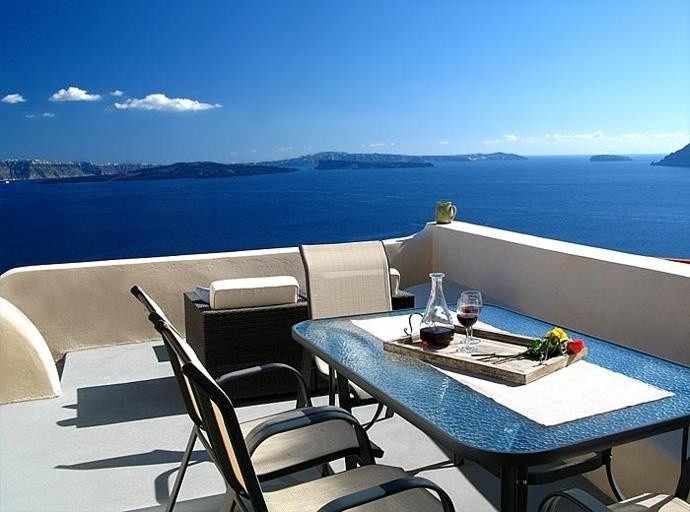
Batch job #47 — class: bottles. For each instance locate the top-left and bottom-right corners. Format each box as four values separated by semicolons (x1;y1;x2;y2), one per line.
418;272;455;349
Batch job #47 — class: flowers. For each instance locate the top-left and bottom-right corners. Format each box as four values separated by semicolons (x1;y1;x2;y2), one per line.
466;323;586;365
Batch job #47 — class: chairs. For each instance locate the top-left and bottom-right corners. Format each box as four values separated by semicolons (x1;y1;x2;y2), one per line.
152;319;456;512
128;285;384;511
291;240;402;426
534;457;690;510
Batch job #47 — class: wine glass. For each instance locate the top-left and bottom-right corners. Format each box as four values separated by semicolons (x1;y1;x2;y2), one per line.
457;289;483;356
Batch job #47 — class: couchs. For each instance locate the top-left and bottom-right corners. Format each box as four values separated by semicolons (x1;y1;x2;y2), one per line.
180;291;412;409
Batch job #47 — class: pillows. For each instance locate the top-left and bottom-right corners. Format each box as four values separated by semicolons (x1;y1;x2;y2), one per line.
192;285;211;304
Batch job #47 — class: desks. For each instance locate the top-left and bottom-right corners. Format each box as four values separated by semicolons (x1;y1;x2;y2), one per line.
289;297;689;510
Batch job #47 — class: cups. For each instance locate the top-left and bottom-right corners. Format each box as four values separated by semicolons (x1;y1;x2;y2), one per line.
435;201;456;224
